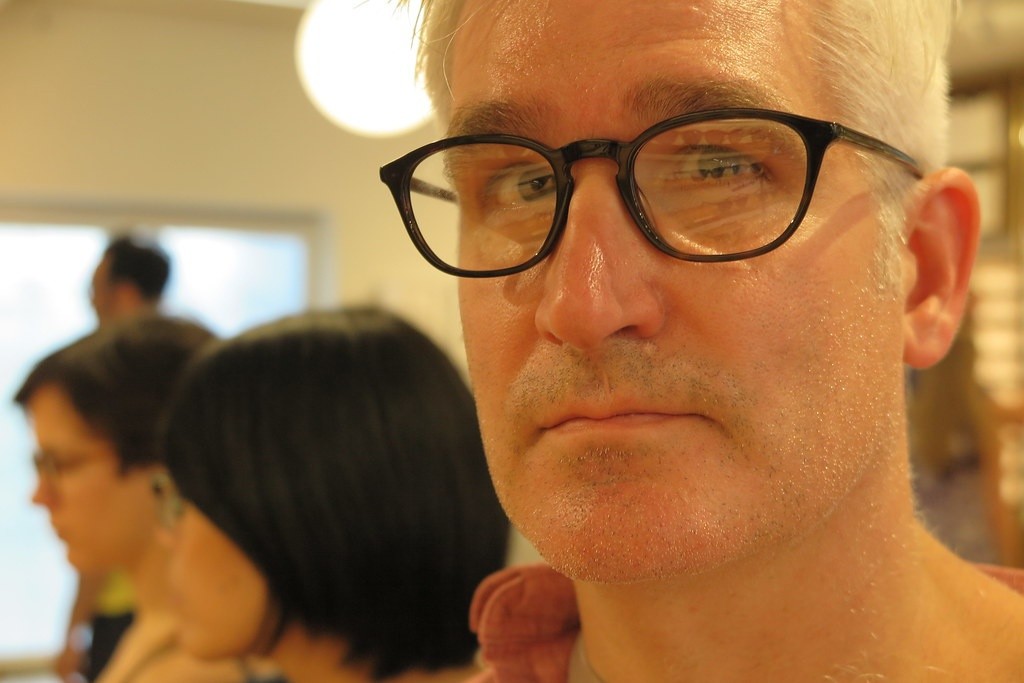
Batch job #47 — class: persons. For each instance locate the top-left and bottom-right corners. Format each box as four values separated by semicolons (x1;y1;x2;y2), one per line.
378;1;1024;683
14;316;221;683
150;306;510;683
57;236;171;683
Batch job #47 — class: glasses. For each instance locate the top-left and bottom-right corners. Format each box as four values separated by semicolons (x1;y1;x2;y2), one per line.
379;107;926;278
33;452;129;481
